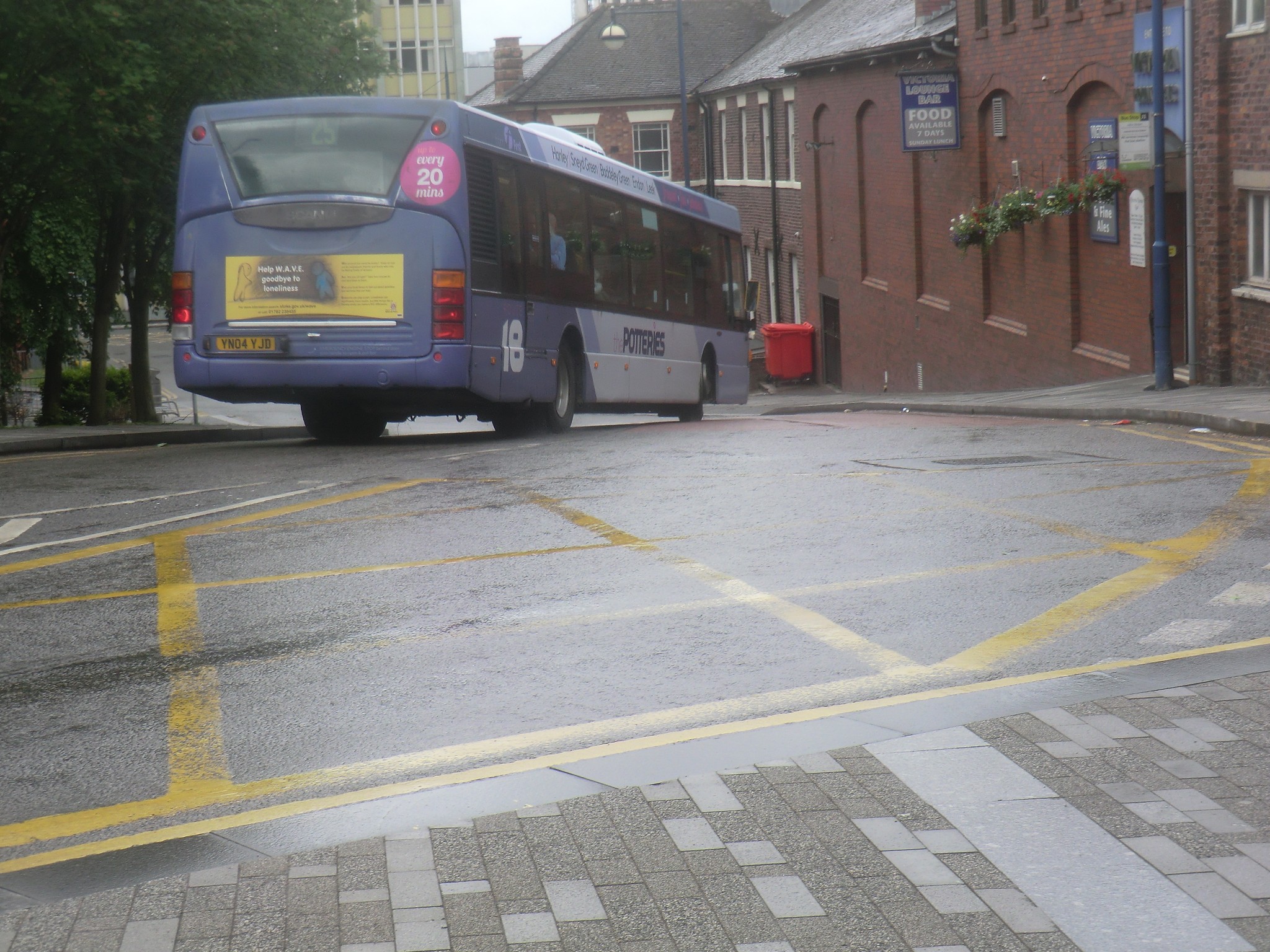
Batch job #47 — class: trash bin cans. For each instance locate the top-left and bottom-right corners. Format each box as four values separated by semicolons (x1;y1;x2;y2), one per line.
760;322;815;387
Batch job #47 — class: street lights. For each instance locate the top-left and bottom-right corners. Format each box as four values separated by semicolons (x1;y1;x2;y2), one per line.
603;2;695;189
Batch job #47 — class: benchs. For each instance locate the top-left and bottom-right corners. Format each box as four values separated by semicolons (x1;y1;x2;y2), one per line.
152;394;180;424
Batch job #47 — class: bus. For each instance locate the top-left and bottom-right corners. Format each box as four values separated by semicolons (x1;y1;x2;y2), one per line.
169;94;760;441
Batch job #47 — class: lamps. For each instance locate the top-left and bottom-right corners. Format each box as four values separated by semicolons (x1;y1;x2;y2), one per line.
804;140;834;152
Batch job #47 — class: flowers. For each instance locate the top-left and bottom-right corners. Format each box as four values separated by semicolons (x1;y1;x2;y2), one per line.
949;165;1132;259
